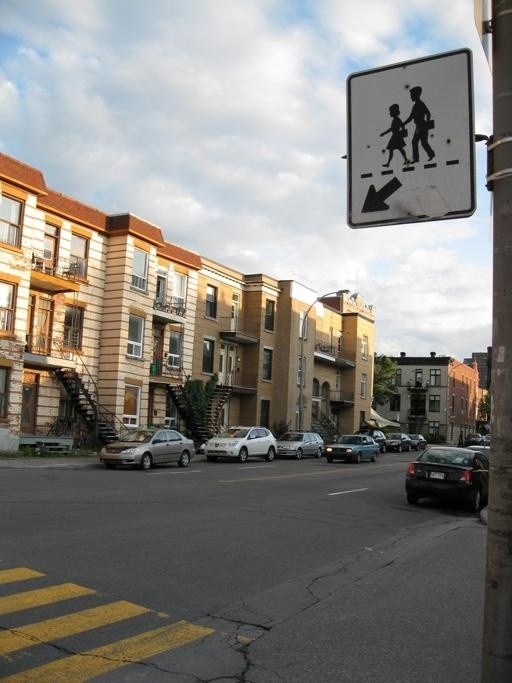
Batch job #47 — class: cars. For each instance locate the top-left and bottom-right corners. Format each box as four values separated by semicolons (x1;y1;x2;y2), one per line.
274;428;491;464
98;428;197;471
404;445;490;514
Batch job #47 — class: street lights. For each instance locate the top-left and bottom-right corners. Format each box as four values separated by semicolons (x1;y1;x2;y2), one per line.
445;360;473;428
298;288;351;430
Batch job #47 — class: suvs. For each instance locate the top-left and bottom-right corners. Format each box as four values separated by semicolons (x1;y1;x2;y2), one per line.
203;425;279;464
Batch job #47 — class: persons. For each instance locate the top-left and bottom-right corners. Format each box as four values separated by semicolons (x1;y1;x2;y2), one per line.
457;430;463;448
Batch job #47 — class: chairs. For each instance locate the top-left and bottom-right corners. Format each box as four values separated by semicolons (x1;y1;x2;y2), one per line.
32;252;81;283
154;294;186;317
